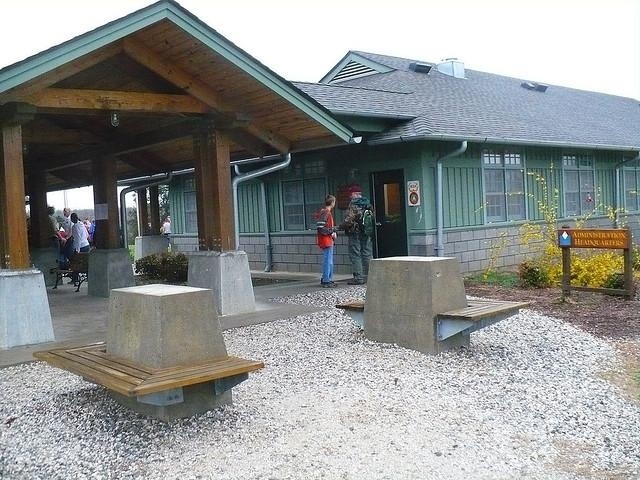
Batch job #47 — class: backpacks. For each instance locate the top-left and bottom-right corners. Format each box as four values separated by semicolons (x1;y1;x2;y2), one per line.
354;200;375;237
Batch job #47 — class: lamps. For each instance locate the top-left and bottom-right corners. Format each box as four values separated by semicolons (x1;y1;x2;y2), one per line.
107;109;122;127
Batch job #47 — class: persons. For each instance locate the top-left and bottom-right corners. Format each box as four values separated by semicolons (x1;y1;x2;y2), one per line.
163;217;171;248
316;194;338;288
45;204;96;271
333;186;375;285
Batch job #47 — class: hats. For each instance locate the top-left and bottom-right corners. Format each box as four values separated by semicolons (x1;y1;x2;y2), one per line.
347;185;362;193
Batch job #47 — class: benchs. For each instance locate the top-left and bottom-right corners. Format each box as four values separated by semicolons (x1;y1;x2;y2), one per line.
47;250;97;294
334;294;531;356
33;335;267;425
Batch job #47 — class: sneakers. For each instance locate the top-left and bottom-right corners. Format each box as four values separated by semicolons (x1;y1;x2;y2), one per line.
348;277;364;285
363;276;367;283
321;283;336;288
